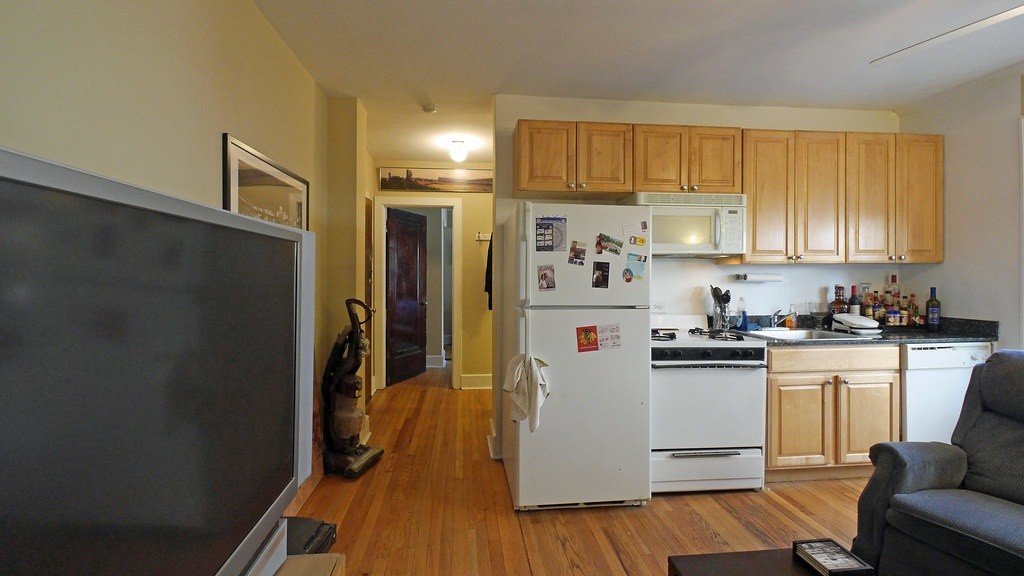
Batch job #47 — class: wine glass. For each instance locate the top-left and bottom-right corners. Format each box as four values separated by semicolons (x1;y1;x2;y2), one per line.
808;301;830;330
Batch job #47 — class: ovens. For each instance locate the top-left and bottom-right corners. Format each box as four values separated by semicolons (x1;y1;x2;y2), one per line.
616;190;746;258
650;356;768;493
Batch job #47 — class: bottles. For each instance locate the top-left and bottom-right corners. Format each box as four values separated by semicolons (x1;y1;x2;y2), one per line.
847;286;861;316
859;274;919;327
828;285;849;323
926;287;941;331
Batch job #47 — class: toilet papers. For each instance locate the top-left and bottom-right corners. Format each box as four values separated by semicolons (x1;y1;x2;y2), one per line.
743;272;784;282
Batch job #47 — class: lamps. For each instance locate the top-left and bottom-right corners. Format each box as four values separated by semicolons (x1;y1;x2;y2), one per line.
449;141;467;162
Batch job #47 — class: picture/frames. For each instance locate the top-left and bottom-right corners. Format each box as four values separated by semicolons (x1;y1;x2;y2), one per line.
378;166;494;193
223;133;310;231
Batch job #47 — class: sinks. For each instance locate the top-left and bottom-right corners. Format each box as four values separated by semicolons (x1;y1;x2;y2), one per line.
731;326;873;342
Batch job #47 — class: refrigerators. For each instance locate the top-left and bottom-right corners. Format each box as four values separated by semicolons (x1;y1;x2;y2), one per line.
500;199;654;511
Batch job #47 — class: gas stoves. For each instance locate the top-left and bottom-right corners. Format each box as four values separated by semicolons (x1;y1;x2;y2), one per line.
646;308;768;368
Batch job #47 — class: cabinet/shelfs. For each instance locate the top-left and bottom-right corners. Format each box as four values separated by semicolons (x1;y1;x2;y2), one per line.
510;119;945;265
763;344;902;480
272;553;346;576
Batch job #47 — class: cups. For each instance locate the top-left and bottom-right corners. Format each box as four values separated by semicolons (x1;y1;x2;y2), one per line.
713;304;738;332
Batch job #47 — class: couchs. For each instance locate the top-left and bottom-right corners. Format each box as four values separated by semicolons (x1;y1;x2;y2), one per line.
850;348;1023;576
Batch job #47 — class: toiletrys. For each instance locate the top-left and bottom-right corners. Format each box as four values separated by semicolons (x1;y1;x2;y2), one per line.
786;304;797;330
736;297;747;331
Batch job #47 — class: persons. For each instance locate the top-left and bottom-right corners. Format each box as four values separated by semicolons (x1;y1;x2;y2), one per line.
624;270;632;282
539;273;554;288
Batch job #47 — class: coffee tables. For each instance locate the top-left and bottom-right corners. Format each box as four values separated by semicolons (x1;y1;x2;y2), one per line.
667;550;817;576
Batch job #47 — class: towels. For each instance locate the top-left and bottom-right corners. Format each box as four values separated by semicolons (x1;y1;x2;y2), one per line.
502;353;549;434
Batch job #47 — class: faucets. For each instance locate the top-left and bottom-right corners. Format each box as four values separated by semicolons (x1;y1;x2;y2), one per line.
770;309;798;327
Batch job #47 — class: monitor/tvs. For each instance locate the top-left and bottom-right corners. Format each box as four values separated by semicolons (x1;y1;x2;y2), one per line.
0;148;316;576
